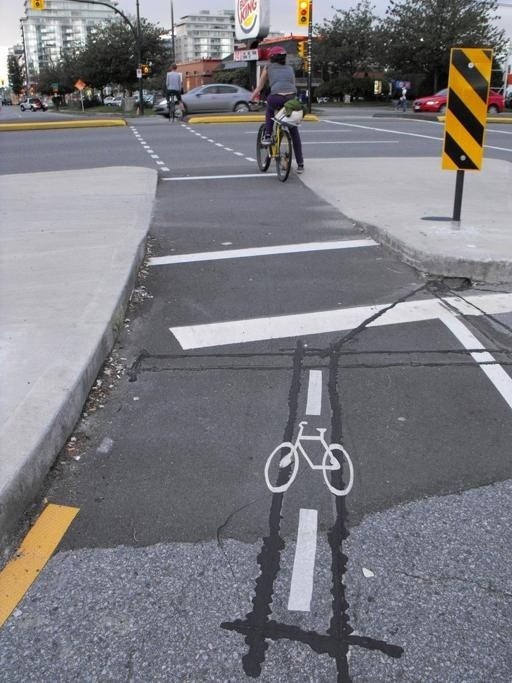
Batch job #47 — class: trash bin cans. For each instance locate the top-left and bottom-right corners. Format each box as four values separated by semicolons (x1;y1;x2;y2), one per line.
345;94;350;103
123;97;134;110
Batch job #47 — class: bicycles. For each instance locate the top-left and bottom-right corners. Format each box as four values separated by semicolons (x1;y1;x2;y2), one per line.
167;91;176;125
247;98;295;183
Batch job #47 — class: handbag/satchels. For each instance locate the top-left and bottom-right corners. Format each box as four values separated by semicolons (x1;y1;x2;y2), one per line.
273;98;307;127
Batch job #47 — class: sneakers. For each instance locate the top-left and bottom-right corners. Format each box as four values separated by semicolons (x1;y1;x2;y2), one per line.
296;165;304;174
261;134;272;145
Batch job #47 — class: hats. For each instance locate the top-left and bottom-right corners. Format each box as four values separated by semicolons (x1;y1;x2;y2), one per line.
268;46;287;56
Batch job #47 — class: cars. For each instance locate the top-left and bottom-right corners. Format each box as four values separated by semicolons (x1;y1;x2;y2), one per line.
20;97;48;112
411;83;506;115
2;97;12;106
151;81;264;119
103;94;154;109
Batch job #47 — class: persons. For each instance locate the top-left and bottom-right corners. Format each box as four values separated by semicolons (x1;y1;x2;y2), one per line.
249;45;305;174
164;64;185;111
395;84;409;112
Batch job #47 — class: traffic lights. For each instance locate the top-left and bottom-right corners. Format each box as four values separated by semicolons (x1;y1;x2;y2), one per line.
31;0;44;10
297;0;310;26
